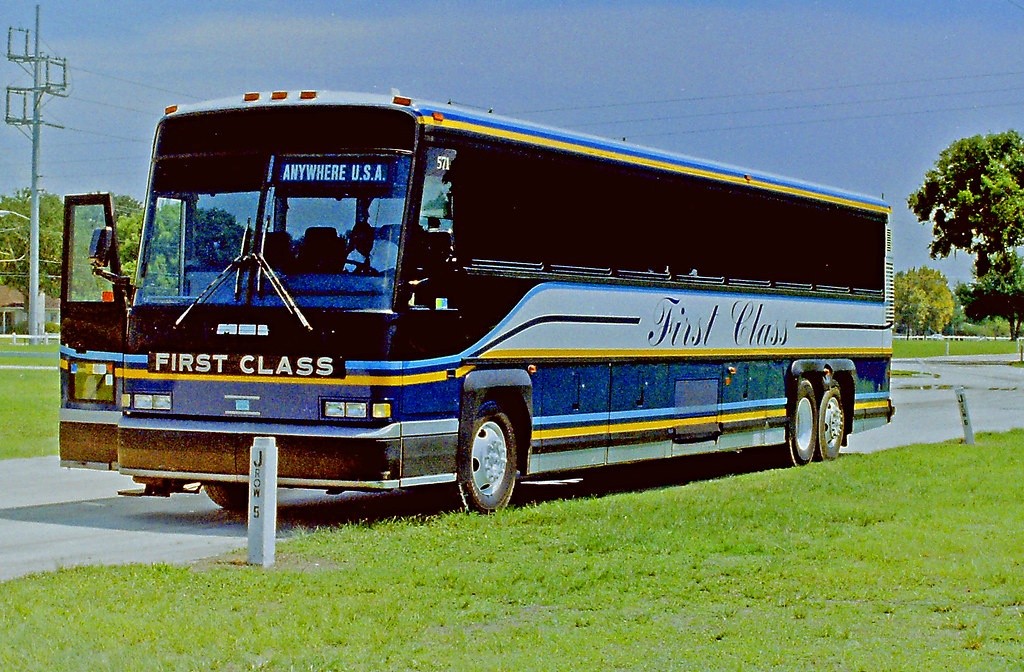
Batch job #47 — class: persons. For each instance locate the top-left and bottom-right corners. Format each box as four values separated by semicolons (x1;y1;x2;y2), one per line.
343;222;398;276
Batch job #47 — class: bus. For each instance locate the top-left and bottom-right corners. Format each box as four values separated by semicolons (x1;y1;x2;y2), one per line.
60;89;895;515
